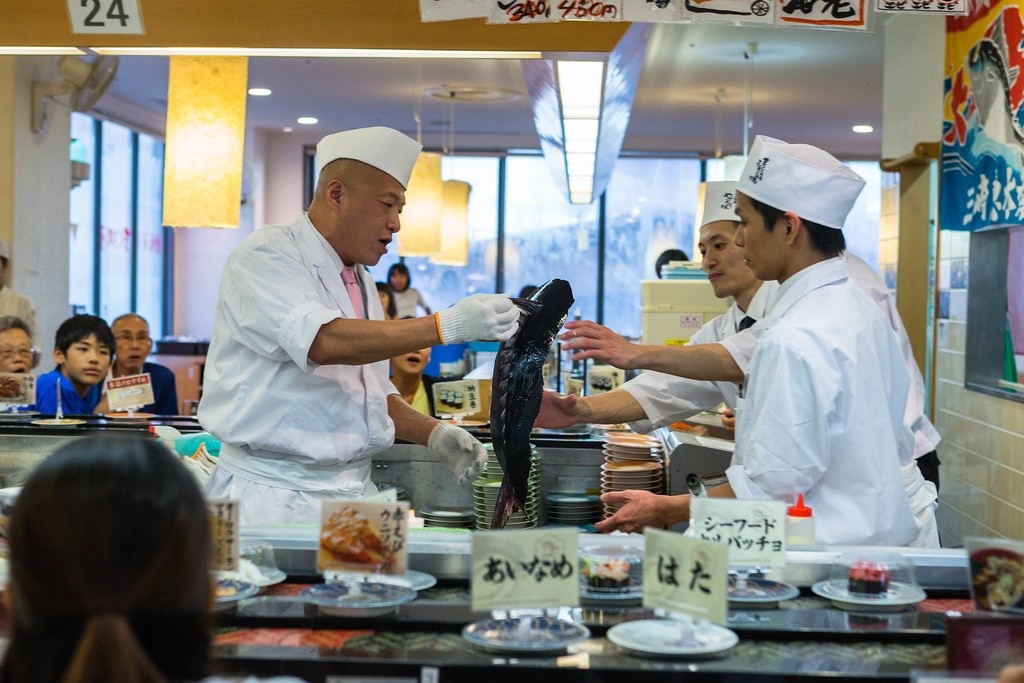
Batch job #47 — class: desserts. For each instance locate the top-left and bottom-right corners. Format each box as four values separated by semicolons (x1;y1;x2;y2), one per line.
846;563;888;592
583;559;629;592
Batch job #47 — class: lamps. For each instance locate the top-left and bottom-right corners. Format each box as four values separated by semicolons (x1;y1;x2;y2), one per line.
398;79;447;258
427;81;522;268
161;54;251;226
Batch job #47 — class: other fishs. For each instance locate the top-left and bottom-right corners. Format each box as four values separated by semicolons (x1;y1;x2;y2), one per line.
489;277;575;528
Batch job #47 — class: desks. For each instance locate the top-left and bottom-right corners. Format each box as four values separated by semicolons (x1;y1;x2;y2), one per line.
142;354;207;416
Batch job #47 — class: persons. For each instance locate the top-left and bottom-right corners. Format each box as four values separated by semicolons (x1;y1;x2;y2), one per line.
386;262;435;317
1;432;221;683
194;125;522;531
534;133;951;549
109;313;183;417
391;314;450;420
0;238;44;369
374;280;400;323
32;314;127;418
0;315;35;374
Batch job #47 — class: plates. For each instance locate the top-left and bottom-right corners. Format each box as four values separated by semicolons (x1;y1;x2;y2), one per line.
206;563;288;615
607;620;738;658
0;413;41;421
727;577;799;610
31;416;86;428
811;579;928;612
380;431;668;535
465;617;591;655
300;569;437;618
105;411;155;422
573;559;645;607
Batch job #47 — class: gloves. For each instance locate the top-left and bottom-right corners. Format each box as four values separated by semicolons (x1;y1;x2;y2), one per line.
433;293;520;344
426;420;488;489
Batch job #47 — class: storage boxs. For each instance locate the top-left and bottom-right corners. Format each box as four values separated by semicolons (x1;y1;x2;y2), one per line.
641;278;736;374
156;341;209;355
661;260;708;279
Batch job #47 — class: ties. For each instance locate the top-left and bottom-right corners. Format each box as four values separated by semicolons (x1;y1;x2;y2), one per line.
341;267;367;322
737;317;753;335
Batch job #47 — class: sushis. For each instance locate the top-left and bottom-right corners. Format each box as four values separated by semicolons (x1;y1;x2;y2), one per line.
591;375;611;390
440;389;463;408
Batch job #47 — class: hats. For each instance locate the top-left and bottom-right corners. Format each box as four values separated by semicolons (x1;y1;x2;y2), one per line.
0;241;10;261
700;181;748;230
311;125;422;190
733;130;867;232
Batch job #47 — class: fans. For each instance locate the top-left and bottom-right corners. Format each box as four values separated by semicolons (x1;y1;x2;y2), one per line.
31;54;118;134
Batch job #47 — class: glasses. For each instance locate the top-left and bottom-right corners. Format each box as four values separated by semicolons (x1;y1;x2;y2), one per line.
0;347;36;362
114;334;154;346
